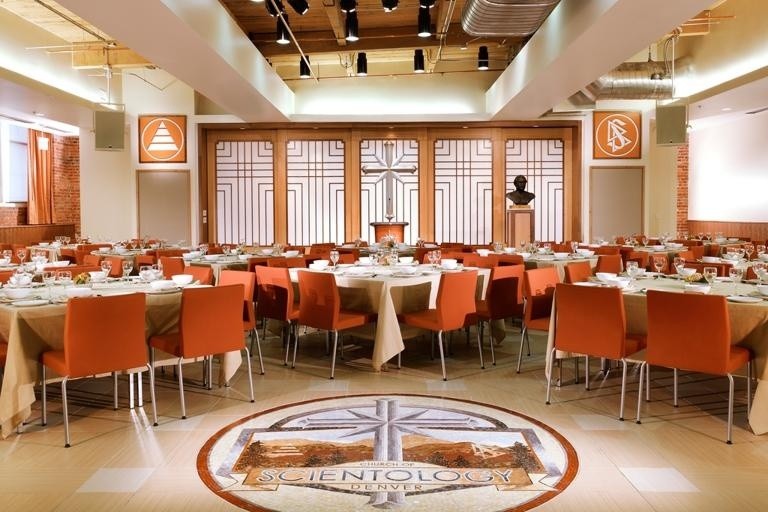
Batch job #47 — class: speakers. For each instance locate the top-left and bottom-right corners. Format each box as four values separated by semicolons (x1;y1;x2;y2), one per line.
95;110;125;152
655;106;687;146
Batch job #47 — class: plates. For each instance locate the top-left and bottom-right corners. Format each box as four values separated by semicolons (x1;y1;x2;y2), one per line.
725;295;763;303
13;300;50;306
572;281;599;287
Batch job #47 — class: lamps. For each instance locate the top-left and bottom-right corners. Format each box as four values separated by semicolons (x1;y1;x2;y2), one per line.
477;46;490;73
262;0;434;79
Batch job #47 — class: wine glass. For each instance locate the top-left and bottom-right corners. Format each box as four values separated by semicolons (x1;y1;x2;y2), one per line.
327;234;445;278
493;229;768;313
0;234;284;311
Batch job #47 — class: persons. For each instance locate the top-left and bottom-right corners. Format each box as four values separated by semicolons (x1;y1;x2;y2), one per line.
505;175;535;206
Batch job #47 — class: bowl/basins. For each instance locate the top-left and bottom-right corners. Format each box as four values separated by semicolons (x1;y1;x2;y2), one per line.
304;241;458;277
0;237;302;306
477;234;768;305
